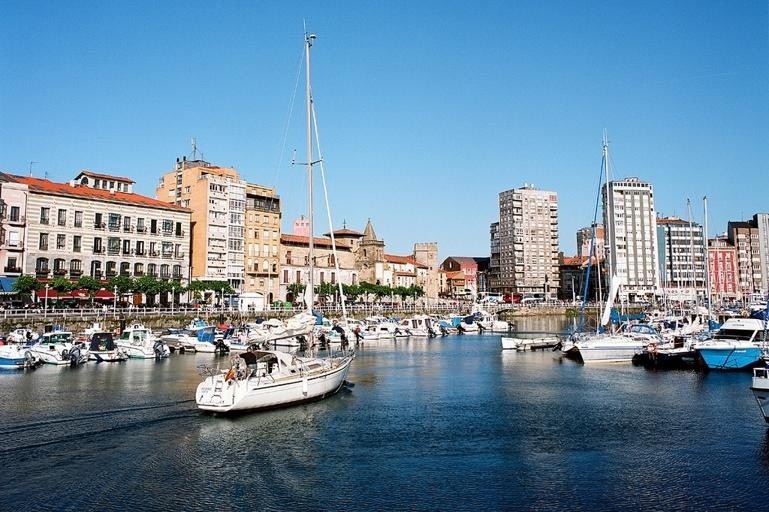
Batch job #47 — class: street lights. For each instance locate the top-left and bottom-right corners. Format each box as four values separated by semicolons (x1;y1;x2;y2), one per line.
302;284;459;309
44;284;48;316
171;286;175;314
113;285;118;317
220;286;224;310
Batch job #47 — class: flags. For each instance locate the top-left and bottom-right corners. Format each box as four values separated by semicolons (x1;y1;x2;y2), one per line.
225;365;236;382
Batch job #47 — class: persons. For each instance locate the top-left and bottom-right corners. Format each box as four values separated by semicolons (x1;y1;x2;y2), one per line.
236;346;257;371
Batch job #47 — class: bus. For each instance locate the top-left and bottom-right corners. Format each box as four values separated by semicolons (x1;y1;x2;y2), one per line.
476;292;556;303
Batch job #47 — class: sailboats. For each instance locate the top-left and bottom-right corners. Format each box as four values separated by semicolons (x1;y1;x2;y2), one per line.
193;17;357;419
